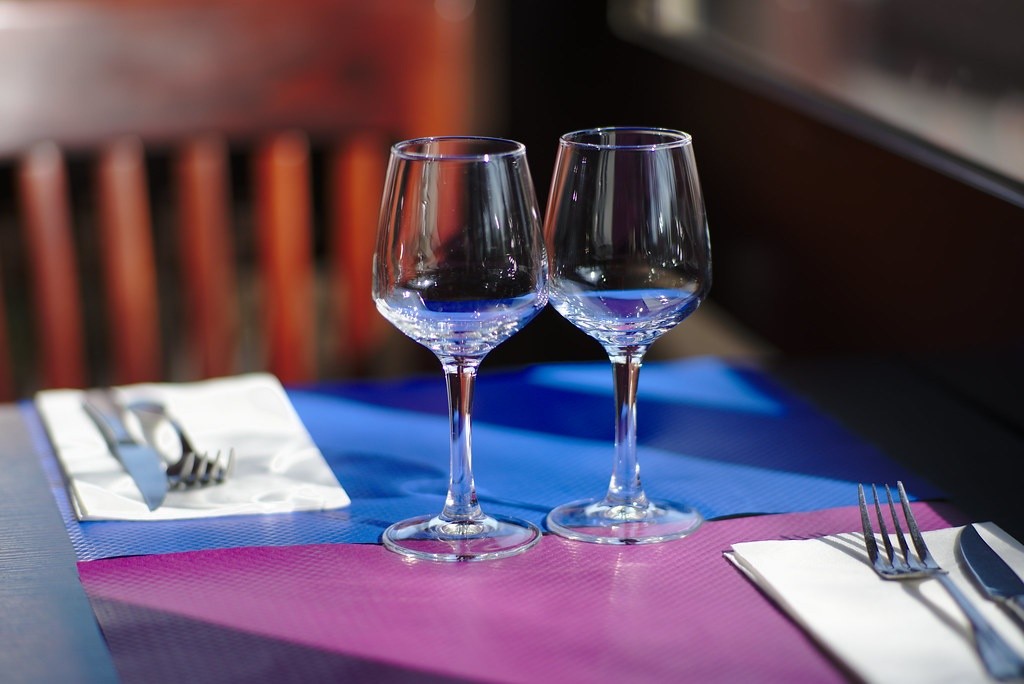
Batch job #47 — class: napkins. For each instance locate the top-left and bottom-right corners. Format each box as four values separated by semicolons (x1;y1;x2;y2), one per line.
33;371;352;524
721;520;1024;684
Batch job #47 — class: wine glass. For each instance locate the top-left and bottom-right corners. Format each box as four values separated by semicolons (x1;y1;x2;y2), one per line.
371;134;547;563
544;125;713;545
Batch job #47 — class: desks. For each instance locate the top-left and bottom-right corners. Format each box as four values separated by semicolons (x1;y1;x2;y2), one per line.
0;355;1024;684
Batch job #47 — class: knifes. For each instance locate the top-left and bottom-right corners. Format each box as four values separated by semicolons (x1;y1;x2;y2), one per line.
959;522;1024;624
80;387;167;511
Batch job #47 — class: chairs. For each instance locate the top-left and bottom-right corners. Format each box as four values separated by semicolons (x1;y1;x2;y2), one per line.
0;0;480;409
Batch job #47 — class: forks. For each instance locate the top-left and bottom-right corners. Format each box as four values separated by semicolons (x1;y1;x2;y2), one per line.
856;480;1024;678
128;396;233;490
